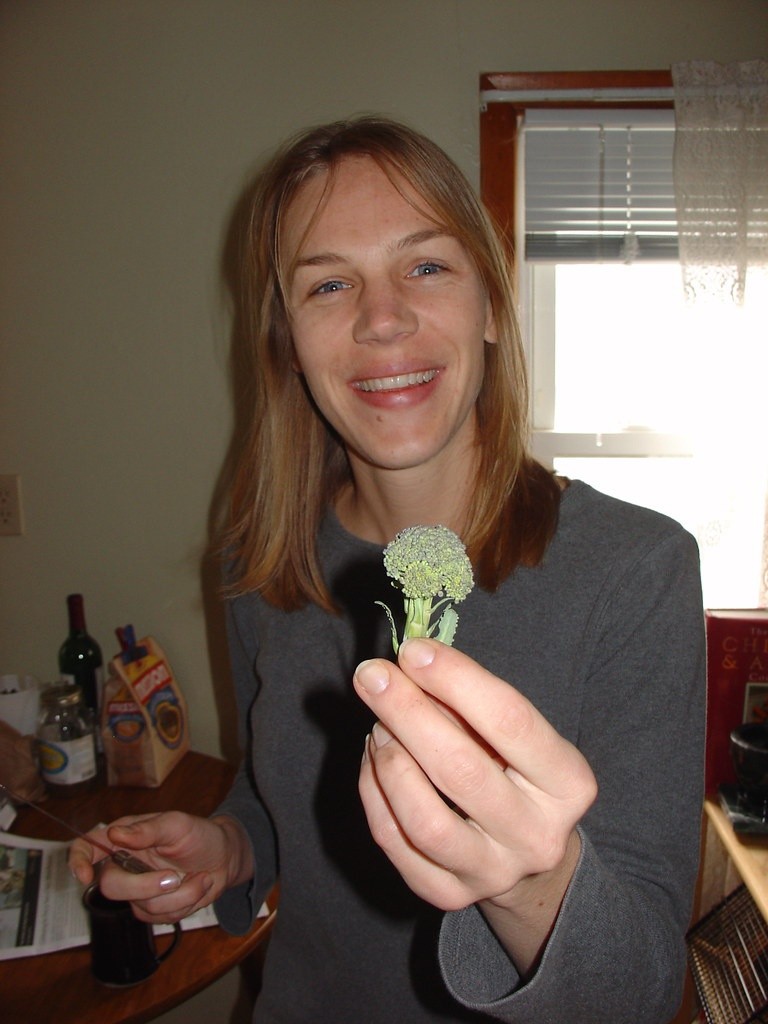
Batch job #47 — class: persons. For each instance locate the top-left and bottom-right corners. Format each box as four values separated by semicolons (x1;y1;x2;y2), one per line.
66;114;708;1024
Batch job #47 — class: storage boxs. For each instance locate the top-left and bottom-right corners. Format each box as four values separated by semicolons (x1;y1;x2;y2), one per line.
686;882;767;1024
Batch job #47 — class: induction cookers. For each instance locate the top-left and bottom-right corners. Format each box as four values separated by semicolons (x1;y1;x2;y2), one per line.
716;783;767;835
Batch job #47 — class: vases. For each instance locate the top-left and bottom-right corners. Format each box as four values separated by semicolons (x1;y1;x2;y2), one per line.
729;724;768;815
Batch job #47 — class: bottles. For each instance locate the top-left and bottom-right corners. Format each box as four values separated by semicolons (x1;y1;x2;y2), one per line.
40;686;101;796
57;594;104;758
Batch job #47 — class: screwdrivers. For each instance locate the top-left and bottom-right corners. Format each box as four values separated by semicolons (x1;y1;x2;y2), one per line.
0;782;153;873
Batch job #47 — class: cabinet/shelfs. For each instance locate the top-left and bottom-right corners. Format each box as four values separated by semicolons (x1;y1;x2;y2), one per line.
692;796;768;1024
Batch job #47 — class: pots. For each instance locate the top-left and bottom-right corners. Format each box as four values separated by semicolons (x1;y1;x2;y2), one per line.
731;721;766;800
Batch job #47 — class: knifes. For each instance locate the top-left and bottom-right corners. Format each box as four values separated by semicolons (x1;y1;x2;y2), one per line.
1;785;155;873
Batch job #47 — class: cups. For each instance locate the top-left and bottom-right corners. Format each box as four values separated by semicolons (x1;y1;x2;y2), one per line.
83;883;181;986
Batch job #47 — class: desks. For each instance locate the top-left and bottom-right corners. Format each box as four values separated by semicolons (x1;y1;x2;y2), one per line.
0;748;280;1023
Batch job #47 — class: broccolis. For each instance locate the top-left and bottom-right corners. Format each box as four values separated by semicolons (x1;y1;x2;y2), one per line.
374;525;473;654
